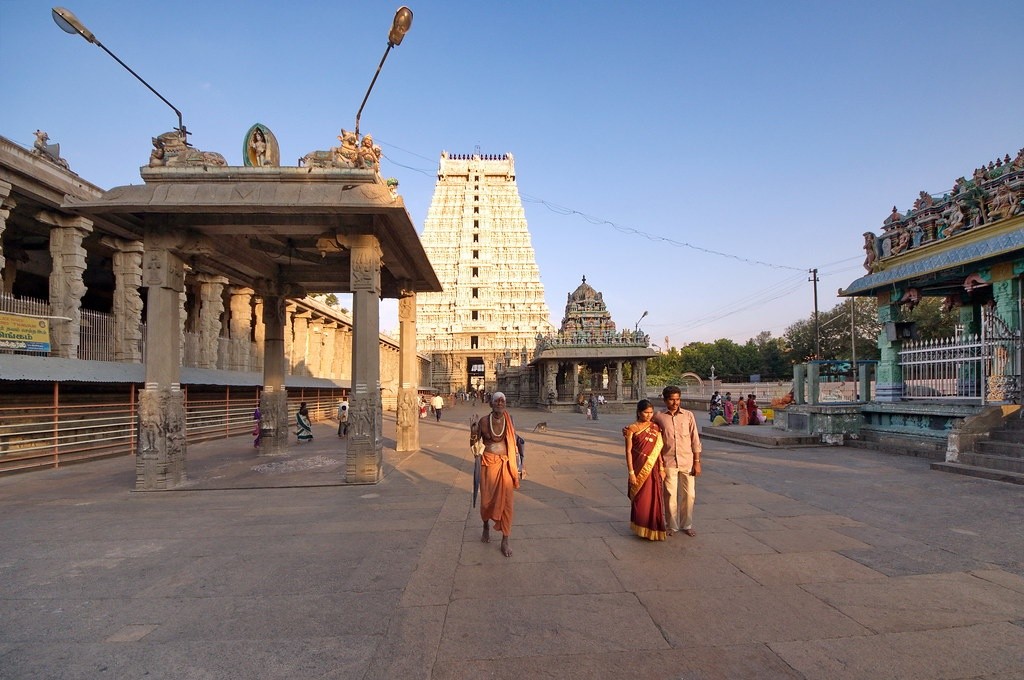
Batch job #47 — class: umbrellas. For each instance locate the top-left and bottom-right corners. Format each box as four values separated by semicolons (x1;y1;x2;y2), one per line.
471;435;485;508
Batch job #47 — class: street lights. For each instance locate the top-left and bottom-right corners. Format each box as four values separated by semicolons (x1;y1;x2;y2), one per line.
631;310;650;400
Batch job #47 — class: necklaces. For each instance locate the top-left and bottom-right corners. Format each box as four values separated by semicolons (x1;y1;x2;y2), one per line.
489;414;505;442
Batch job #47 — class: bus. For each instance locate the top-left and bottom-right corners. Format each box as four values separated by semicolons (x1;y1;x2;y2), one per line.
808;359;880;382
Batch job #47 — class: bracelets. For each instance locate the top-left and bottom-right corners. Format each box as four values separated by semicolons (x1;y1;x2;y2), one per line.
629;470;634;475
694;460;700;462
659;466;664;470
659;461;663;464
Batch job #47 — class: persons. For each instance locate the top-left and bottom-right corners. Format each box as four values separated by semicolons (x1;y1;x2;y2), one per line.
622;386;701;541
338;397;349;437
469;392;516;557
450;389;491;406
296;402;313;441
250;130;266;166
417;391;444;421
575;391;607;420
252;401;261;448
710;391;767;426
